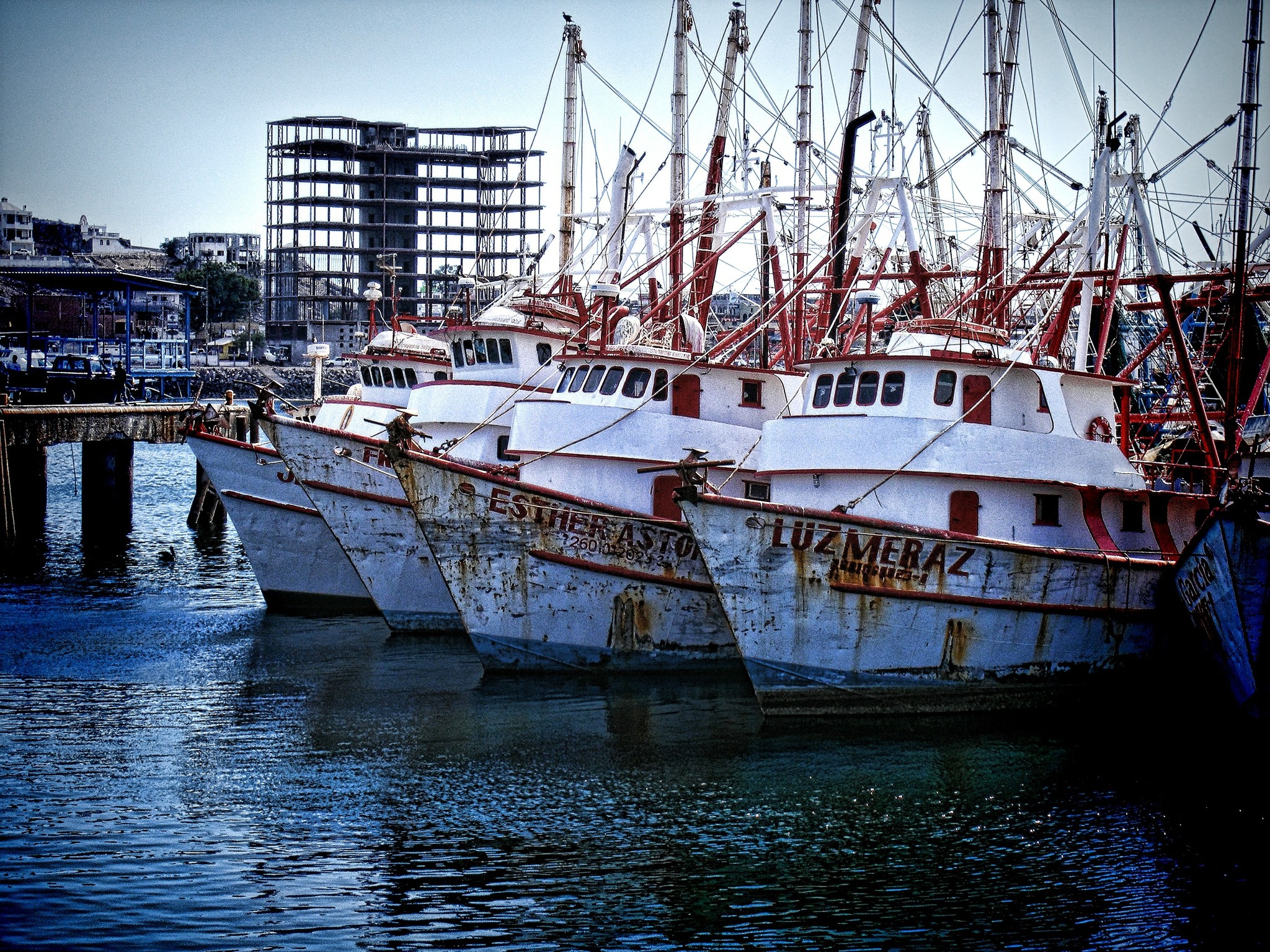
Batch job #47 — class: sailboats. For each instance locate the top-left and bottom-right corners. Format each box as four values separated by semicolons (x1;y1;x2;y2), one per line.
185;0;1270;727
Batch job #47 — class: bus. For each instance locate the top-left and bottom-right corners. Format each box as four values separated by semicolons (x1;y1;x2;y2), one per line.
8;336;96;369
98;339;188;369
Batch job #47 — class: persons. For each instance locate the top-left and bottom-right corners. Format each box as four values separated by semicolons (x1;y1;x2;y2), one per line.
112;361;129;404
7;354;21;400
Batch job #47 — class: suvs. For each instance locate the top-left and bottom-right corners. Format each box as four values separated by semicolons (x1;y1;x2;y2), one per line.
323;356;352;368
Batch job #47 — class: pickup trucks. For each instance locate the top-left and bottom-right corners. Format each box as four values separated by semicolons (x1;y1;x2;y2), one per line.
6;354;135;405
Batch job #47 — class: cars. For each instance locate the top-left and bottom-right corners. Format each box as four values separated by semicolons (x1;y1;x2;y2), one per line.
12;248;32;257
181;348;218;367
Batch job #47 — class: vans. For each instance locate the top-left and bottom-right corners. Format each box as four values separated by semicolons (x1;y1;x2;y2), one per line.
24;350;53;368
0;349;35;369
